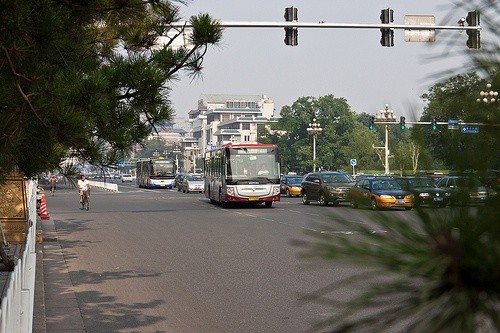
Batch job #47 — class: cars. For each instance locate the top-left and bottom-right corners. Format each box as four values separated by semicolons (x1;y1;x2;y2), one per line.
85;171;133;183
348;170;500;211
279;173;303;198
175;172;205;194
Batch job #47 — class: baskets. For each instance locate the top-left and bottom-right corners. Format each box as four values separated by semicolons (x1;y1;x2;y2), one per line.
50;183;56;187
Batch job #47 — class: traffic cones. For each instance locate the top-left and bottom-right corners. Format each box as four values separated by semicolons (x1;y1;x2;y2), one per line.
38;196;49;220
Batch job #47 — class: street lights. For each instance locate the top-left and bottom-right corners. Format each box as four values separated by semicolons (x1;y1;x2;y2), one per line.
377;103;397;175
307;118;322;172
477;84;500;121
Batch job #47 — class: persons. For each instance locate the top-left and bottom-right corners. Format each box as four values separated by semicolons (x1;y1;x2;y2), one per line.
49;176;58;193
77;175;91;209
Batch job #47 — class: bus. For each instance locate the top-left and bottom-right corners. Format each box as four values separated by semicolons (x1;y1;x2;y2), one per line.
202;143;282;208
136;158;178;190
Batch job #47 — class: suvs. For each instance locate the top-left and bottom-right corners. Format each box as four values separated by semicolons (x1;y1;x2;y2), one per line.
299;171;358;207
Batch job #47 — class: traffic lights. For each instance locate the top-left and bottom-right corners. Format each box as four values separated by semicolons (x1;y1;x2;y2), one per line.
380;9;395;47
466;11;481;50
432;116;437;130
400;116;405;130
369;117;375;130
284;7;300;46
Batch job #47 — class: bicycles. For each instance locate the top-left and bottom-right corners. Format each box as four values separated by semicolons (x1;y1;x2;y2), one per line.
79;187;91;211
49;181;57;196
